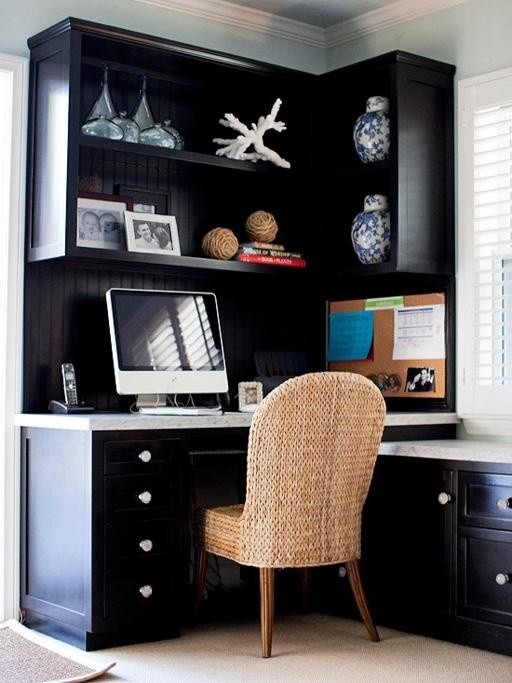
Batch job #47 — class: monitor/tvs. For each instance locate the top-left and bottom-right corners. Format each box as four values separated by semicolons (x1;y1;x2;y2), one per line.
105;287;229;411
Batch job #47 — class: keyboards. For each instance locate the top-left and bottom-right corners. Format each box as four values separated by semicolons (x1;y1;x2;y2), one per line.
139;406;217;415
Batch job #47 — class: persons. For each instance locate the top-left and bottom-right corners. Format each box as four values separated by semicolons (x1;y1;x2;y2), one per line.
376;368;434;392
79;211;172;250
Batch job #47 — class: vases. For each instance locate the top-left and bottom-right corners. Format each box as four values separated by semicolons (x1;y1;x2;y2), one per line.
350;92;391;166
349;192;392;266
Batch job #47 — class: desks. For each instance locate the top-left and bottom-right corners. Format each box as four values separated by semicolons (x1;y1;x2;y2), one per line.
16;400;463;657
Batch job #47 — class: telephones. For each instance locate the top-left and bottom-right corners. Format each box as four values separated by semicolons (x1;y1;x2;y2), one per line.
49;362;95;414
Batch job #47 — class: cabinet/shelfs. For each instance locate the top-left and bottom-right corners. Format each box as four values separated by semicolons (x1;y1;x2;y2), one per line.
311;46;457;282
444;460;512;661
315;443;464;645
21;13;320;282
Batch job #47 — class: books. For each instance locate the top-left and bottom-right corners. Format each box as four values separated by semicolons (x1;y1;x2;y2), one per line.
233;241;306;267
138;405;224;416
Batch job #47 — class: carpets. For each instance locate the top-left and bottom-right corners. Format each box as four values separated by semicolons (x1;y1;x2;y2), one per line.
0;625;118;683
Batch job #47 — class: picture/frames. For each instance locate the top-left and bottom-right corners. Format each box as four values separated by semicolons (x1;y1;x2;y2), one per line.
75;188;134;253
120;208;182;257
111;181;173;221
236;380;264;414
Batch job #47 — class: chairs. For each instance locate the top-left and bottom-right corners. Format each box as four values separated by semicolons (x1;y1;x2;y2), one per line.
189;368;392;661
